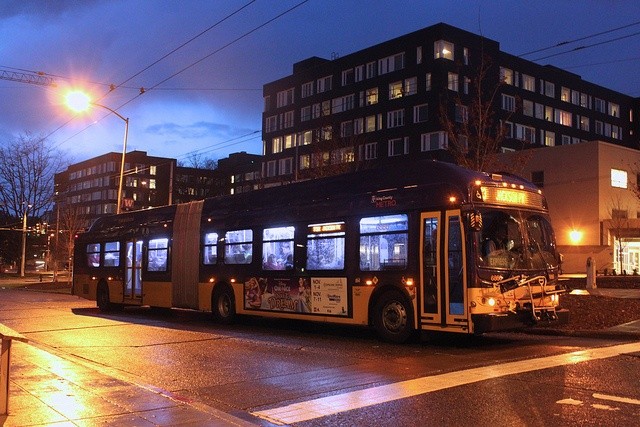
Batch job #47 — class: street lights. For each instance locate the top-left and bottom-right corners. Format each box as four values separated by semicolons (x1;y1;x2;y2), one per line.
20;202;33;277
63;88;130;215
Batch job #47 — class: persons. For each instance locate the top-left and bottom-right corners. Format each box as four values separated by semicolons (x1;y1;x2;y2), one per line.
264;254;285;270
297;278;307;313
246;279;260;307
483;226;508;253
88;246;100;267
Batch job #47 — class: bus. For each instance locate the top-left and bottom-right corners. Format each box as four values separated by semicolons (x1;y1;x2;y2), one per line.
71;159;574;345
